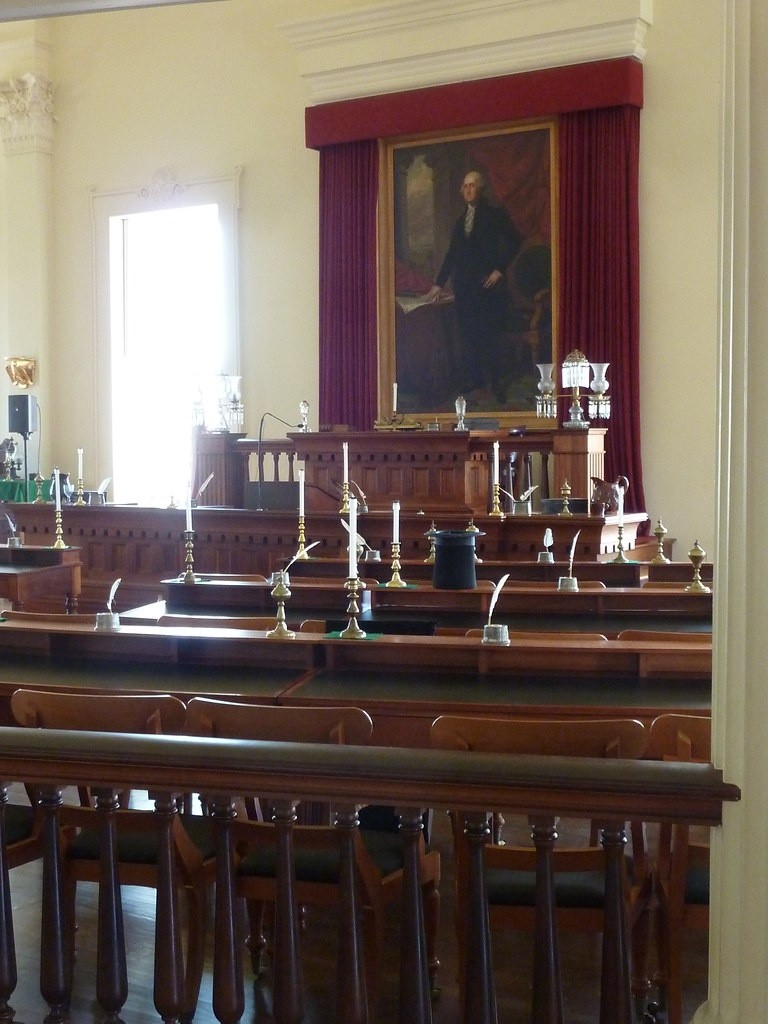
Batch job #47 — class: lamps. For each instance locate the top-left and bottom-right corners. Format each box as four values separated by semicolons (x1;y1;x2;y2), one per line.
533;346;612;428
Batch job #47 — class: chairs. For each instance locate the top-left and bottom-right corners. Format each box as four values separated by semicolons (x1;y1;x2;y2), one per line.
3;558;721;1021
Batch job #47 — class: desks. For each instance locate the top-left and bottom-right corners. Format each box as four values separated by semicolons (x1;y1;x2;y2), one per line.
1;656;310;733
434;610;699;640
278;673;709;759
115;599;434;636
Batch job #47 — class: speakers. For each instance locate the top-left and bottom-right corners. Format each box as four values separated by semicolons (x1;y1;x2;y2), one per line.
8;395;38;433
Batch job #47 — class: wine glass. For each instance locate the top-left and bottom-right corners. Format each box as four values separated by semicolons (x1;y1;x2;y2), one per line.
63;484;74;505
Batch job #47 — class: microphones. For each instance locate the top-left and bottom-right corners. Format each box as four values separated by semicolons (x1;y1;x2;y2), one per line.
258;413;303;510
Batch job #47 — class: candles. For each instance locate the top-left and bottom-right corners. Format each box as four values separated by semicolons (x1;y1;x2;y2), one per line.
492;440;502;485
185;487;193;530
53;469;62;512
77;446;86;482
343;441;350;486
616;485;626;527
391;502;401;541
347;495;360;578
298;467;306;514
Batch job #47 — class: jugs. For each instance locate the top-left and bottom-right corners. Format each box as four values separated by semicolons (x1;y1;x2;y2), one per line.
591;474;629;517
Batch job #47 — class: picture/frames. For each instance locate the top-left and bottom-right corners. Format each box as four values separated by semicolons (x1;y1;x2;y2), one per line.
375;120;562;430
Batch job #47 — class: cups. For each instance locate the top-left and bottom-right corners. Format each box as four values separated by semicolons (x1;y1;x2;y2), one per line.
590;503;609;518
